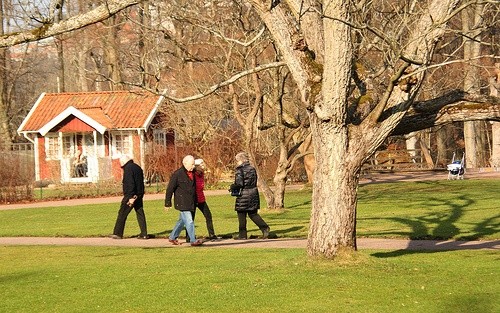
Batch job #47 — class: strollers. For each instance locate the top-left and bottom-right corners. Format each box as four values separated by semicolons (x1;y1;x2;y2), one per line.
448;151;465;181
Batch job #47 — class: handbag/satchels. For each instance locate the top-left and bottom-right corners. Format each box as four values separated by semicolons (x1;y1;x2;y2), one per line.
231;187;243;196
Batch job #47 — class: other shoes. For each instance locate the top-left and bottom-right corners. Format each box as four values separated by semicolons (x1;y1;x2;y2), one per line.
168;237;182;246
234;236;247;240
262;226;270;239
186;237;197;242
190;240;204;245
136;234;149;239
210;236;223;242
109;234;122;239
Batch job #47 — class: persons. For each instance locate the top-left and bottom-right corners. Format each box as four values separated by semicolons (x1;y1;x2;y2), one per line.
109;154;147;239
186;159;223;243
165;154;203;247
75;150;88;178
228;152;270;240
